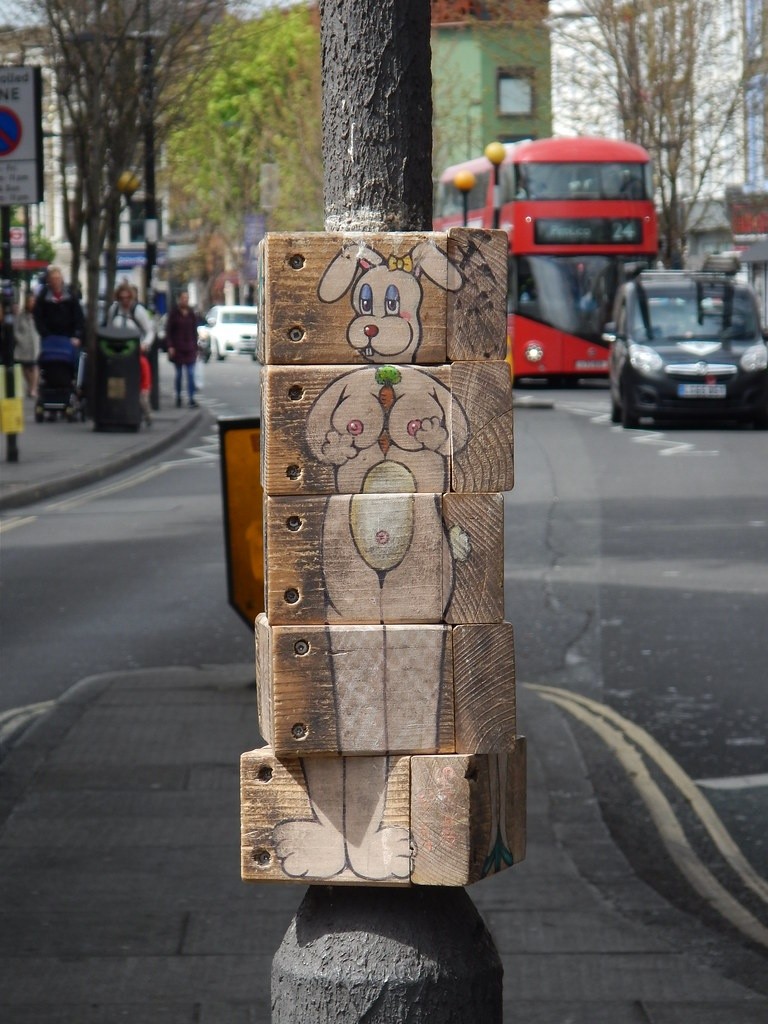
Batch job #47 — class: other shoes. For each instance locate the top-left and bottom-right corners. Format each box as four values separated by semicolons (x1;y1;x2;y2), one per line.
176;397;181;406
189;400;199;409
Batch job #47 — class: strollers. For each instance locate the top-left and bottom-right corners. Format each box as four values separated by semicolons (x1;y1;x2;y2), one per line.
34;336;87;423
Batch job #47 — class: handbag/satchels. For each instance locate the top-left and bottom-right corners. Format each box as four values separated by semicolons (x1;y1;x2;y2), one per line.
160;337;169;353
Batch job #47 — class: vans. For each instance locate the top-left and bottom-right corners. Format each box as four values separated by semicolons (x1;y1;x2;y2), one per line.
602;270;768;428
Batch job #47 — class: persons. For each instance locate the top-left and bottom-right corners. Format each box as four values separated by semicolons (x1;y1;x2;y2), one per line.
17;266;217;426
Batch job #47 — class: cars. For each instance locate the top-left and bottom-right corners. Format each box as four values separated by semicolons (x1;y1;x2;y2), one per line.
197;305;259;363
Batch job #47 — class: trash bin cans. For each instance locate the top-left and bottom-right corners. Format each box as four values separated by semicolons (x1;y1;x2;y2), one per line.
94;328;142;430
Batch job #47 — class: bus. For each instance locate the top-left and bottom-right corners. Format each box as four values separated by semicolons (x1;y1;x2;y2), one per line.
432;136;658;388
